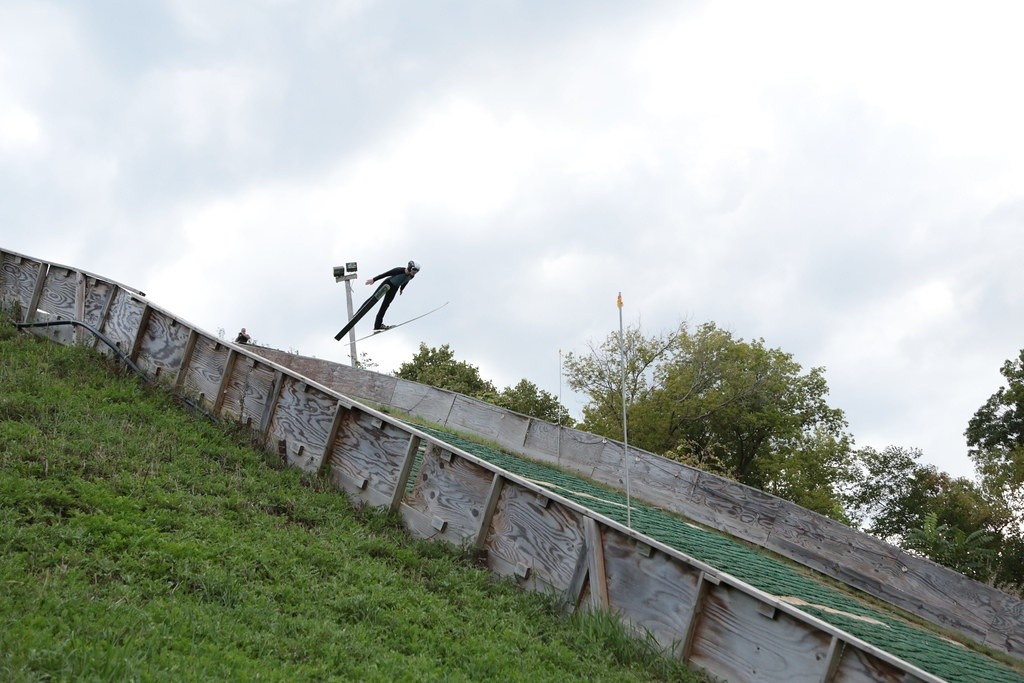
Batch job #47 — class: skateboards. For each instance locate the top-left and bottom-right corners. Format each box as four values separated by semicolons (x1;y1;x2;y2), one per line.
343;301;451;347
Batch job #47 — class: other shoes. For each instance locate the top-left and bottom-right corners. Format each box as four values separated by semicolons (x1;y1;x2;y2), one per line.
374;324;386;329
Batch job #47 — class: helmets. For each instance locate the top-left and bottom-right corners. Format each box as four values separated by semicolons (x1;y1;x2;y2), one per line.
407;261;420;272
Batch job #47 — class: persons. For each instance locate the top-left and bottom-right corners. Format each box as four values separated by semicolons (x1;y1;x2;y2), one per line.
355;261;420;330
235;328;251;343
